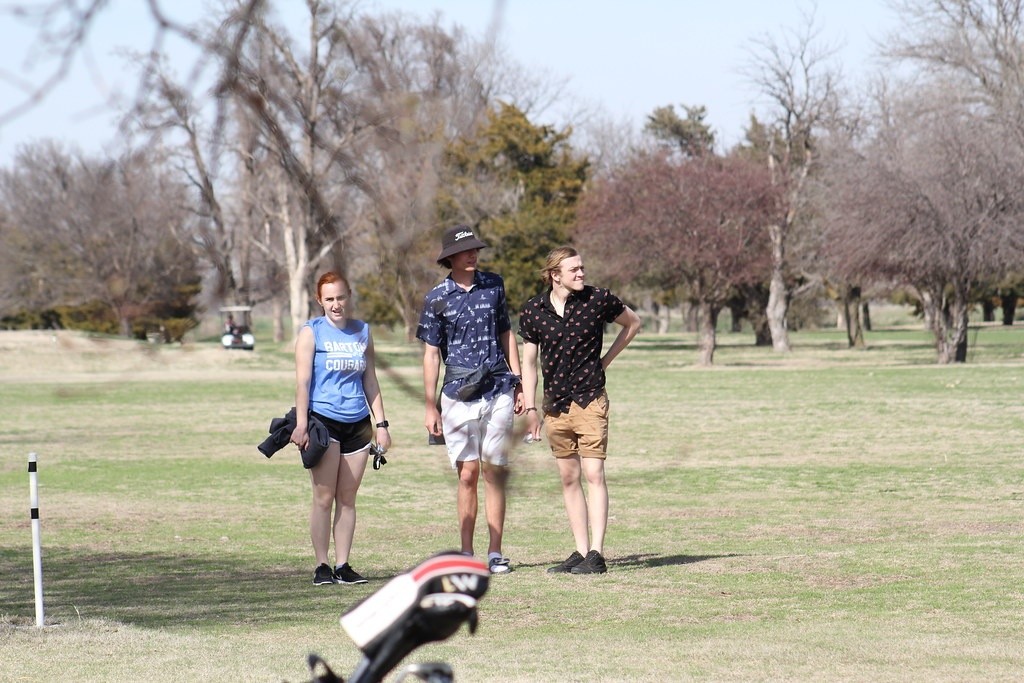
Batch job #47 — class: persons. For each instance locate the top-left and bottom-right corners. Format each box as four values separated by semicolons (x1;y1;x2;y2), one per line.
517;246;643;575
415;225;525;577
290;273;391;586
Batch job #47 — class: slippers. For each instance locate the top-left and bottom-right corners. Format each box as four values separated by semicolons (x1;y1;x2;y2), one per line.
488;558;511;575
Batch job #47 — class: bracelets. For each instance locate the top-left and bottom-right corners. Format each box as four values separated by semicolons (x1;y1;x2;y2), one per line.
525;407;538;415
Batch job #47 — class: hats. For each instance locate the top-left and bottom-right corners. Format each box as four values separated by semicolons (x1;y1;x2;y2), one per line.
436;225;488;261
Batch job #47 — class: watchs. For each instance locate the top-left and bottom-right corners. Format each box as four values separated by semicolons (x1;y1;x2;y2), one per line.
376;420;389;428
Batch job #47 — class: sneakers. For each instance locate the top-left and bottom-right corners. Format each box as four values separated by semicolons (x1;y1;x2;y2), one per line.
333;565;370;585
572;550;607;574
548;552;585;573
313;563;335;585
